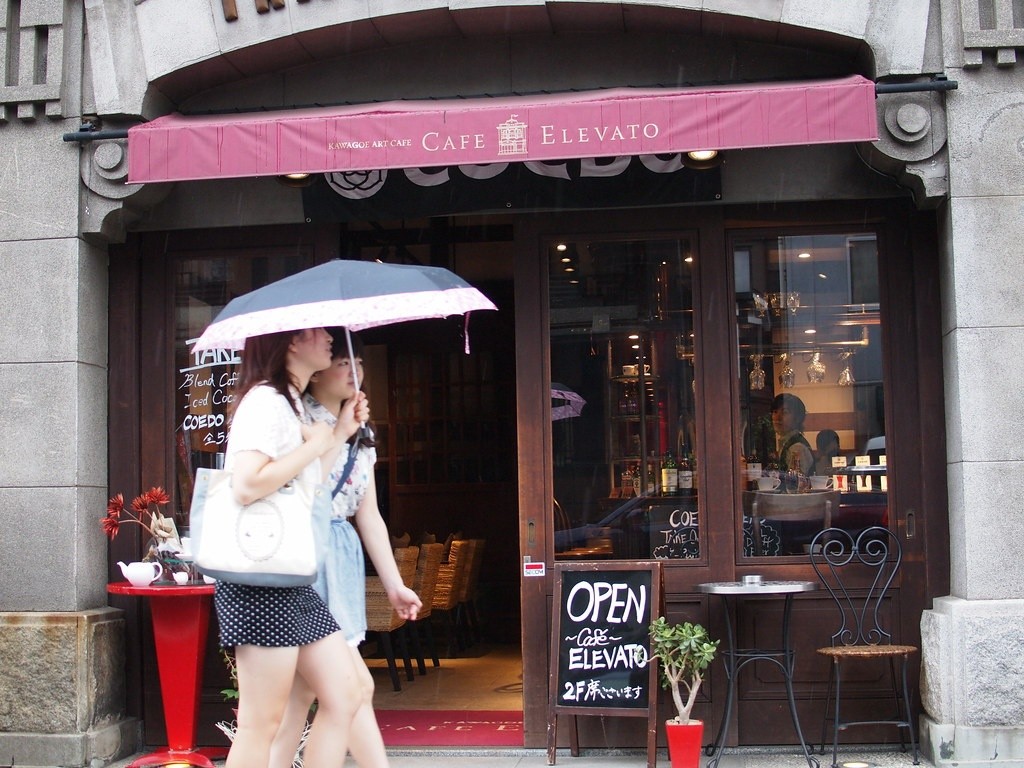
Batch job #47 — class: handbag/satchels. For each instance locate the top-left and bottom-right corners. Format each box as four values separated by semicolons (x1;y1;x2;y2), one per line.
187;381;320;588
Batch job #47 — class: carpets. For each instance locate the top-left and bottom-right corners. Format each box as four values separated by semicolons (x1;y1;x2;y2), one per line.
372;708;523;747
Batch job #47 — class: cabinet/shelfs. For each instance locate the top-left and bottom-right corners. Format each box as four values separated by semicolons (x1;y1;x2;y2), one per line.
600;342;658;467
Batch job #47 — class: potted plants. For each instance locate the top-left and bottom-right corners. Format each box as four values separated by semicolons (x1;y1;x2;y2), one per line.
645;617;722;768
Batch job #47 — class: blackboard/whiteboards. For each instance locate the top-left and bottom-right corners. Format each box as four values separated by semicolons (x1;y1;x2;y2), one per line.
641;498;781;559
548;562;666;718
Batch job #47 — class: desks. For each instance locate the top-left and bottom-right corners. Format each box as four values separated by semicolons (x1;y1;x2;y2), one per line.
700;580;820;768
104;581;233;767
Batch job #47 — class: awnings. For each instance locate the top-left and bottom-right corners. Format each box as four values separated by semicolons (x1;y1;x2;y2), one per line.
123;71;884;183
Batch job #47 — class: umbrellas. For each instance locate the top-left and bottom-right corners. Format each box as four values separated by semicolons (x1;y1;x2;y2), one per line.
191;256;498;458
551;381;585;420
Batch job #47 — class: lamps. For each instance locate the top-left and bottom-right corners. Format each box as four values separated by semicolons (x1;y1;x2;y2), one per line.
679;151;723;168
274;172;318;187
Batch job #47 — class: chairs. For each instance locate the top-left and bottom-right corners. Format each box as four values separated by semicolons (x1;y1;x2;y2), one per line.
743;490;840;556
360;530;486;692
548;496;613;560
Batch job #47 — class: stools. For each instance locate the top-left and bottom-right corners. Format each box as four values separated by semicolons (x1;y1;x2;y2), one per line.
810;527;920;766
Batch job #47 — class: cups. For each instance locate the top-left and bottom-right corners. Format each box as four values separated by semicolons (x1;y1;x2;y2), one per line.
623;364;651;375
173;572;188;583
809;476;834;488
803;544;820;554
756;477;782;489
203;574;216;584
181;538;191;554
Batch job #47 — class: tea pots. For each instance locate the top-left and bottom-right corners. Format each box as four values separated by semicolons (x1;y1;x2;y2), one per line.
116;561;163;586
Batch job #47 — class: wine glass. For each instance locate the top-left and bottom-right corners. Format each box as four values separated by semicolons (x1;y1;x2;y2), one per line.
838;351;856;386
753;292;801;318
778;352;795;389
749;353;767;389
807;352;826;384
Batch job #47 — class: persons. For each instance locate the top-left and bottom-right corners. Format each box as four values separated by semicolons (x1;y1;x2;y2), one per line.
771;393;848;481
214;326;422;768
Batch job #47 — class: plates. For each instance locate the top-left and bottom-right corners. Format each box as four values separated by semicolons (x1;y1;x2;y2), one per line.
175;555;193;559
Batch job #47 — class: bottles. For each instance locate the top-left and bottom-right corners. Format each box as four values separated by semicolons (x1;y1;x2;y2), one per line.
620;443;699;496
617;381;654;415
740;440;809;494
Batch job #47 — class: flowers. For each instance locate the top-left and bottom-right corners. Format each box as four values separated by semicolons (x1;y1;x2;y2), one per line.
102;486;183;574
